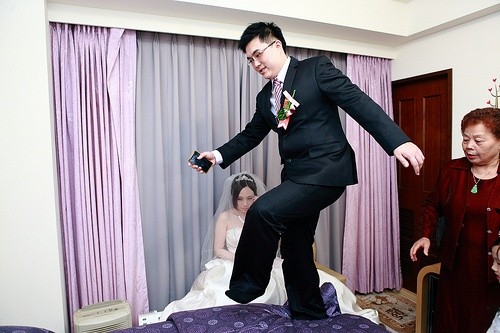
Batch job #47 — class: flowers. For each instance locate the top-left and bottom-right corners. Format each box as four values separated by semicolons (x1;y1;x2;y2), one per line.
275;88;299;130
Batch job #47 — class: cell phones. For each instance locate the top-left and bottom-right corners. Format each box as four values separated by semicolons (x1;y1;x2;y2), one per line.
188;151;213;173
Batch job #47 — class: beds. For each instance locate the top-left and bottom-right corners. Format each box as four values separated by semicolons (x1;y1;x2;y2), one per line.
1;283;394;333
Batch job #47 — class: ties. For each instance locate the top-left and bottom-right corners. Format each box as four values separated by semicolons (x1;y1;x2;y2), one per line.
273;78;283;114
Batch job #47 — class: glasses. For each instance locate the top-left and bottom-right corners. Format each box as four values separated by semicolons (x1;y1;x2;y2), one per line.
247;41;276;65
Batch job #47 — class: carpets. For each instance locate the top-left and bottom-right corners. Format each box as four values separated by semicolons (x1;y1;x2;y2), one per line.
357;292;417;333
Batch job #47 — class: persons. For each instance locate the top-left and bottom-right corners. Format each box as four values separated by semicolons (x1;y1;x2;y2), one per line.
490;234;500;285
186;20;426;320
406;107;500;333
193;171;336;311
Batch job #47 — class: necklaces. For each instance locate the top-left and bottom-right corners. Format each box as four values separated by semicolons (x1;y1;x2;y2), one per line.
471;166;498;194
236;211;246;223
